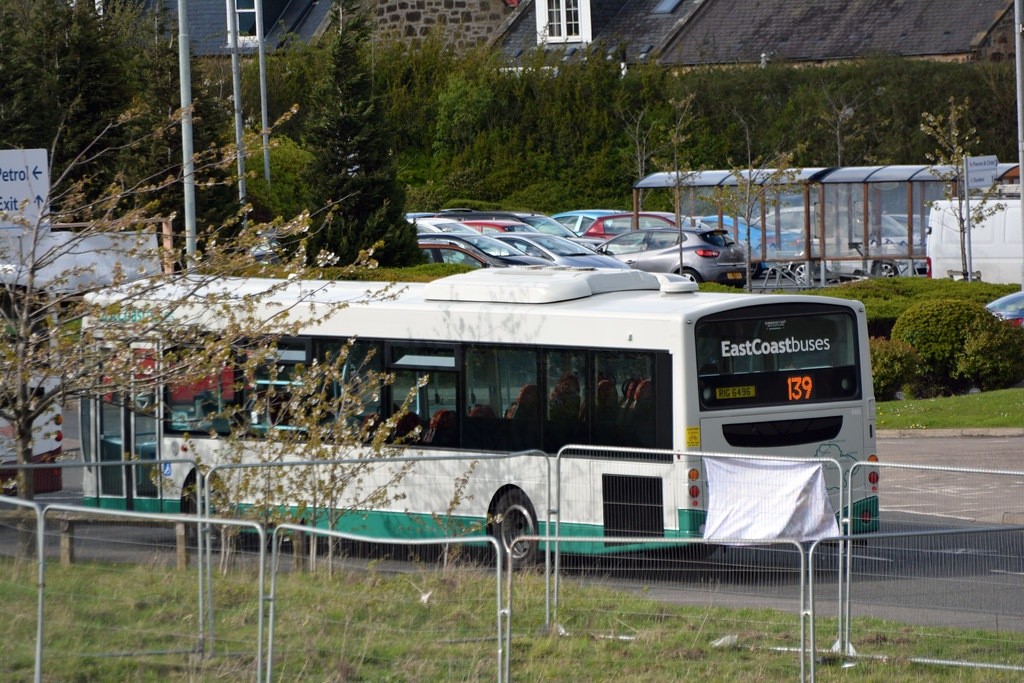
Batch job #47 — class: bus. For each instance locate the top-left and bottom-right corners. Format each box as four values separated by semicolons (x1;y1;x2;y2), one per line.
0;269;72;494
77;260;879;571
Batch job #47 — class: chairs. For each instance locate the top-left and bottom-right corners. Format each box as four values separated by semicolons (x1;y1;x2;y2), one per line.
625;378;655;427
361;413;381;443
506;384;536;421
468;404;497;419
393;412;420;444
424;408;455;444
620;381;637;422
550;371;581;422
579;380;619;426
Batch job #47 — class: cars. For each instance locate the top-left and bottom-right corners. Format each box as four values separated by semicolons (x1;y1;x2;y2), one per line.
401;210;705;277
986;290;1024;328
698;215;815;278
789;222;927;285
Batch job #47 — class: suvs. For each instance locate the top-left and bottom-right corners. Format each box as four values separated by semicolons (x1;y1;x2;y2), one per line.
591;226;748;287
751;206;913;260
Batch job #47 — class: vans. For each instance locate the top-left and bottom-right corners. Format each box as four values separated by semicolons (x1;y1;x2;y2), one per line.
927;197;1024;287
